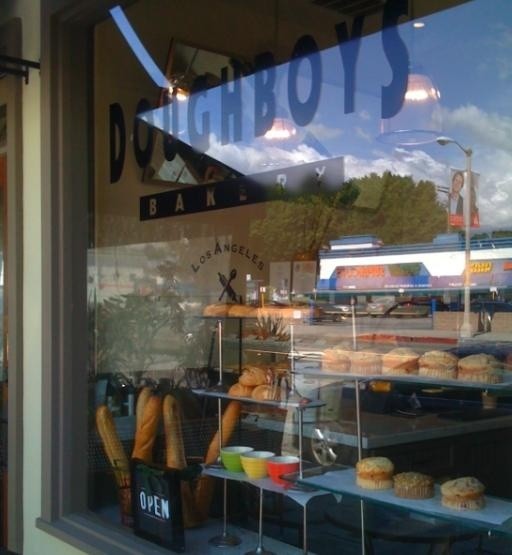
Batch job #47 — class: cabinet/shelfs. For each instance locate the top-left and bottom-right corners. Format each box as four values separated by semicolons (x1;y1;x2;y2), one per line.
185;259;512;553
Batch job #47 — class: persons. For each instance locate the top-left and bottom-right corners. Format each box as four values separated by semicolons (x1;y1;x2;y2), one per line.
471;187;479;224
449;172;463;216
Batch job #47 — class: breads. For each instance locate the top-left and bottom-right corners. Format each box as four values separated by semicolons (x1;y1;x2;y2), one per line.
380;345;422;377
356;457;394;490
394;471;434;500
97;302;386;510
441;476;486;509
456;353;504;385
417;351;461;380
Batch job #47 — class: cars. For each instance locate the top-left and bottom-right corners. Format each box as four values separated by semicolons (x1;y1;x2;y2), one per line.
308;303;343;321
365;298;396;317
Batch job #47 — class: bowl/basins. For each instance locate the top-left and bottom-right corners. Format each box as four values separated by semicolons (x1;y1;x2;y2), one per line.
221;447;301;486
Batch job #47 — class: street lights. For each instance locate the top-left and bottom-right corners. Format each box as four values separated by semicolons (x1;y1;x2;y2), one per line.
290;252;305;301
435;136;473;338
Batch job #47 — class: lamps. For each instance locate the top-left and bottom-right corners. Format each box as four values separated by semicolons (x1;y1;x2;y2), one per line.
378;1;445;148
256;6;297;141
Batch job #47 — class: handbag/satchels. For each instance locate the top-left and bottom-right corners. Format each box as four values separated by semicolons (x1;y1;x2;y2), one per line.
131;457;185;554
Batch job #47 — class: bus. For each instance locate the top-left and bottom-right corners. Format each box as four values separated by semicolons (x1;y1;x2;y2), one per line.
304;285;511;312
